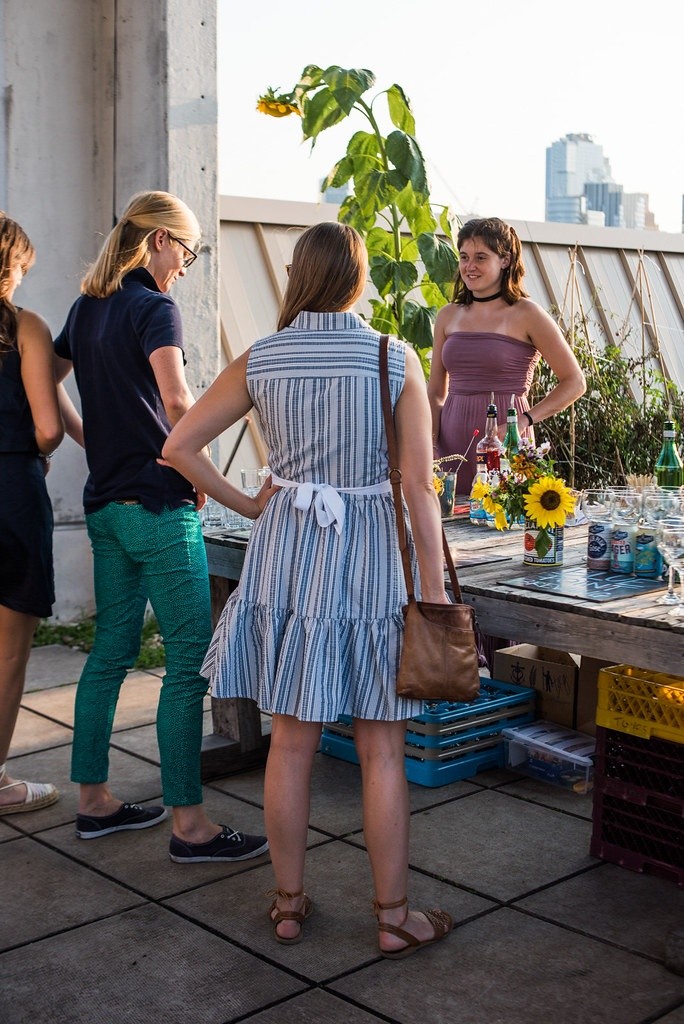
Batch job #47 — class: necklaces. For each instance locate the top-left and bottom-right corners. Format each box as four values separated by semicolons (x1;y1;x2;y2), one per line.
471;290;502;302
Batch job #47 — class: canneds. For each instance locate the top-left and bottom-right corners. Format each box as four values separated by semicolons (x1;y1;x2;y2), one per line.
587;520;684;583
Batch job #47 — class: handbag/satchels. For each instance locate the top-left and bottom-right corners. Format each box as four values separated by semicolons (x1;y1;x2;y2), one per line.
396;596;483;702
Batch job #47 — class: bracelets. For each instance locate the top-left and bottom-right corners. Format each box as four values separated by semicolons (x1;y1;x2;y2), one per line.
523;411;533;427
433;445;438;450
40;452;54;464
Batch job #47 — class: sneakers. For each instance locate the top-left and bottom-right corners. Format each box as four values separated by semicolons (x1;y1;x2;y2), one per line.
76;802;166;840
170;823;271;865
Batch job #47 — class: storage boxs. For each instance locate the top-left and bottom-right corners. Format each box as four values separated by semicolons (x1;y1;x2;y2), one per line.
316;641;684;887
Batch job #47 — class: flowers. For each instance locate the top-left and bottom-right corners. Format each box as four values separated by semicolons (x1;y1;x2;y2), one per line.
467;436;579;533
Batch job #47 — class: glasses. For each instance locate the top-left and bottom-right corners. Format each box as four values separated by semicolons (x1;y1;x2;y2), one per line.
154;229;197;268
285;263;292;276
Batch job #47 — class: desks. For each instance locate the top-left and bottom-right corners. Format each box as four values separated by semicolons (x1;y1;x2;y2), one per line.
202;492;684;790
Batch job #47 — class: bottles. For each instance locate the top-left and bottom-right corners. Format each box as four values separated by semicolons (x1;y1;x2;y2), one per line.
469;473;525;530
476;405;504;485
653;420;684;493
499;408;522;476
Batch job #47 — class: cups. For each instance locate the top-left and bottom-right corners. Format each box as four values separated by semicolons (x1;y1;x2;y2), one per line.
565;489;583;527
199;465;271;531
436;471;457;518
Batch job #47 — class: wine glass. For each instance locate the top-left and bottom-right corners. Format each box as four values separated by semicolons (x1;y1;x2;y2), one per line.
582;485;684;617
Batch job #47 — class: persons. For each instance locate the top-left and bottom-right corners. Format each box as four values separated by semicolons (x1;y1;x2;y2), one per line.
427;217;587;498
0;213;67;820
52;190;268;864
161;220;455;961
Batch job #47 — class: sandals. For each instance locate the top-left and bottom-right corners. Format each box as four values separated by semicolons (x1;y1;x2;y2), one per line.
266;887;314;946
0;762;60;817
372;896;455;960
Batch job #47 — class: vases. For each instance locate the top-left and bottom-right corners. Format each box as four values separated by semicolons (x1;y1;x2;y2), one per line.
521;514;565;567
